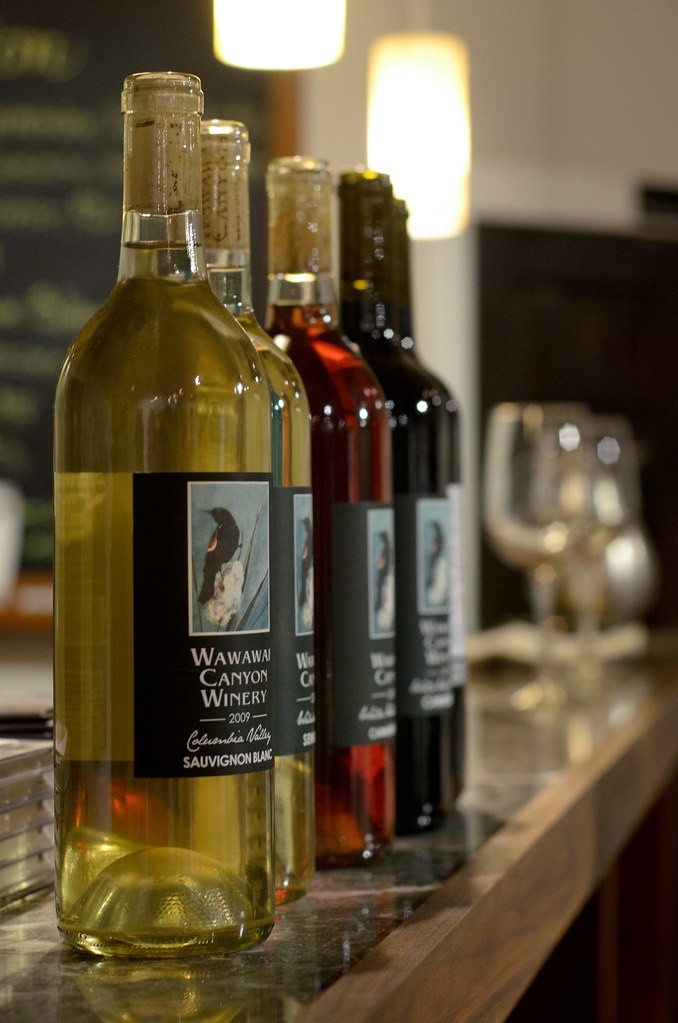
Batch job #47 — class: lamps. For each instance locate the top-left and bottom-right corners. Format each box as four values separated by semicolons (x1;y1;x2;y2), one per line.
363;28;476;241
213;0;347;75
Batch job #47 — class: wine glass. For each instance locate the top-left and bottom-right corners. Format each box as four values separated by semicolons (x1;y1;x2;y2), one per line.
482;402;654;775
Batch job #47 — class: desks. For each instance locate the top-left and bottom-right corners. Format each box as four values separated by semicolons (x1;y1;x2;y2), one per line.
1;620;677;1022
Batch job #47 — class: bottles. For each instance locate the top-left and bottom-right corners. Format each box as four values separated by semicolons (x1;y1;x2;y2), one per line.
263;157;395;870
336;170;452;837
195;118;312;907
392;200;468;810
52;71;276;963
